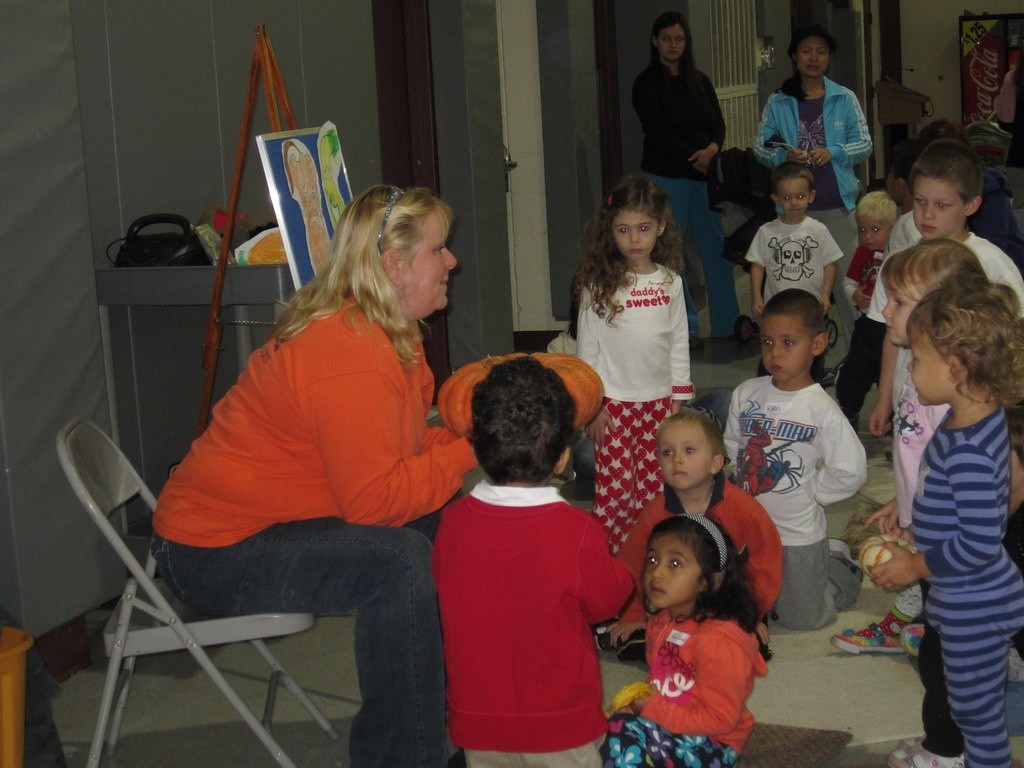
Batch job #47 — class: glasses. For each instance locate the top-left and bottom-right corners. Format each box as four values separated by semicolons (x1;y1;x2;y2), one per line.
378;184;406;255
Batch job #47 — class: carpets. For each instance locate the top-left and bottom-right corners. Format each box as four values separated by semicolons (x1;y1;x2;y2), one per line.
728;724;854;768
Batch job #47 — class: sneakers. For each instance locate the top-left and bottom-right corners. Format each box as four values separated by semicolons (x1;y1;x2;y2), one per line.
829;538;863;581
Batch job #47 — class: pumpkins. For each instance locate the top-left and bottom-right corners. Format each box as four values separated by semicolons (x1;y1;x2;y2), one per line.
439;352;604;435
860;535;922;576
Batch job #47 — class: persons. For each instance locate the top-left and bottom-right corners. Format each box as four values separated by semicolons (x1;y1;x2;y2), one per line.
149;186;479;768
829;119;1024;768
753;24;874;350
601;512;768;768
744;161;844;382
546;272;734;498
834;190;899;435
721;287;868;630
592;410;783;660
431;357;635;768
631;10;740;351
576;174;694;553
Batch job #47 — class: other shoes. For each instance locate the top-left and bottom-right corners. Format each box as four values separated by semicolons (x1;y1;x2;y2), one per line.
617;640;647;662
830;624;907;655
886;748;964;768
595;618;629;650
688;336;703;350
897;736;927;748
711;333;736;343
900;625;926;656
1006;647;1024;683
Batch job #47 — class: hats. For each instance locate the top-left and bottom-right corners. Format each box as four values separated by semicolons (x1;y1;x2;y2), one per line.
787;25;834;60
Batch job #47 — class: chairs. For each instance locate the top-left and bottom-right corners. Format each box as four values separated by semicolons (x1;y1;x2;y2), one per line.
58;415;340;768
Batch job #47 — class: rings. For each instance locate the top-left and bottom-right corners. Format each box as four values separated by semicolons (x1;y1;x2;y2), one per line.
819;159;821;161
